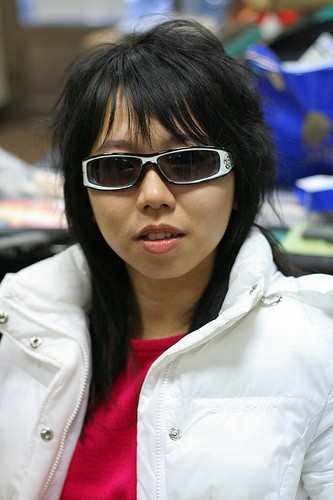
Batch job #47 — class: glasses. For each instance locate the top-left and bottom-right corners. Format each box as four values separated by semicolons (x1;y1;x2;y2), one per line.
81;146;235;191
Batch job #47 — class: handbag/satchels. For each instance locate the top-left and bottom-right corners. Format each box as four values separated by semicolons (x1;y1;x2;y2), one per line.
245;46;333;187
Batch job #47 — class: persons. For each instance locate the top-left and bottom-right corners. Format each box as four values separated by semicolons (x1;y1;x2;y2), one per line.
0;19;333;500
